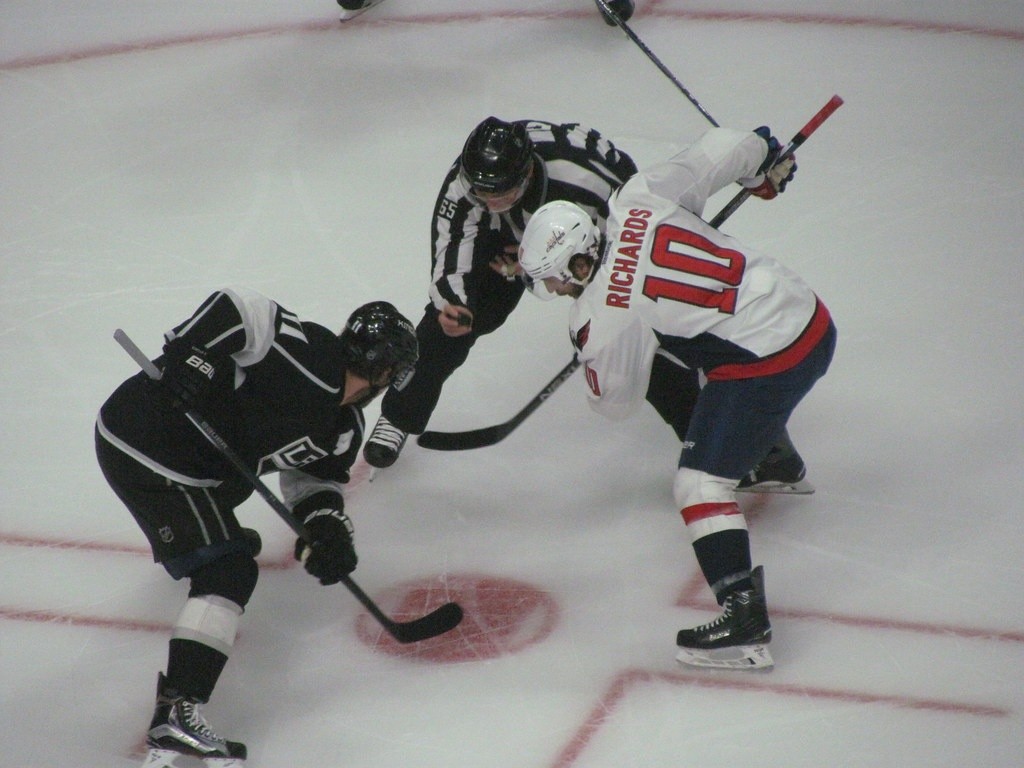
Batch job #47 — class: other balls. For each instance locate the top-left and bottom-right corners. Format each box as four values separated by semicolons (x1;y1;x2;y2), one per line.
446;311;473;328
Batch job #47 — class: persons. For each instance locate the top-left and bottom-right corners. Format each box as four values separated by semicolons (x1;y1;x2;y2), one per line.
336;0;635;27
364;116;837;669
94;287;420;768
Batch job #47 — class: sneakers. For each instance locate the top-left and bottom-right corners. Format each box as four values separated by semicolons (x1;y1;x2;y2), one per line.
735;439;816;493
144;671;248;768
676;566;776;671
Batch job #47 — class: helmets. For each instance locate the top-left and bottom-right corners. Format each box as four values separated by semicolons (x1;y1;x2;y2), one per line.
518;201;602;285
460;117;534;194
340;301;421;380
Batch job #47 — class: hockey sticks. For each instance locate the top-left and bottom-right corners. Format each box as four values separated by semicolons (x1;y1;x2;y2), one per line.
597;0;720;128
113;328;464;644
415;94;845;452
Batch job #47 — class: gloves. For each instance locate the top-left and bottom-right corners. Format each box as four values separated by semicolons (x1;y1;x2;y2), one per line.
147;338;226;424
295;500;357;585
734;126;797;200
